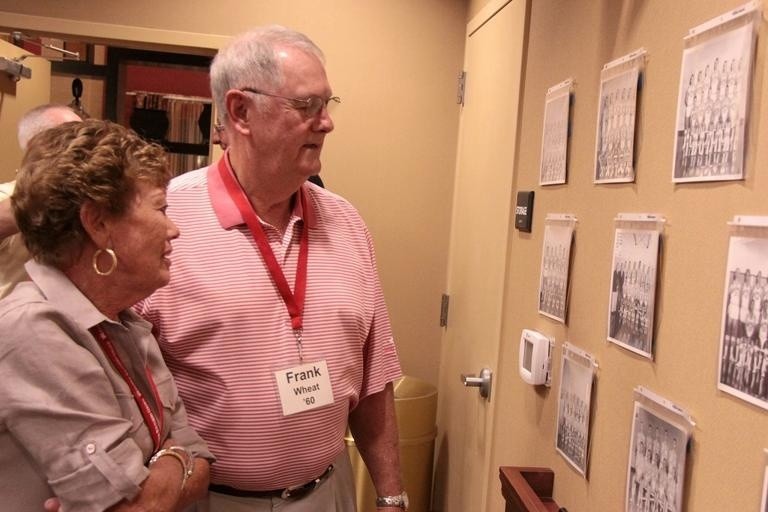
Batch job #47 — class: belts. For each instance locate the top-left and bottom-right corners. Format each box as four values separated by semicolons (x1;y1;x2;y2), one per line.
208;464;333;499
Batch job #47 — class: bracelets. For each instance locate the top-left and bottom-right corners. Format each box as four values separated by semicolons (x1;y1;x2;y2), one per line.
170;446;195;477
148;448;187;490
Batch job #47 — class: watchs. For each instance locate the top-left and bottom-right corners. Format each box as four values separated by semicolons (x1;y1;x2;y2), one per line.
376;492;409;510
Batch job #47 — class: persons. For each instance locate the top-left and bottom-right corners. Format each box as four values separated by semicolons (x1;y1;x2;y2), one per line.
539;244;570;320
557;390;589;472
609;260;653;354
721;267;768;399
0;120;217;512
632;421;677;512
0;105;83;243
596;87;634;178
682;57;746;177
129;25;409;512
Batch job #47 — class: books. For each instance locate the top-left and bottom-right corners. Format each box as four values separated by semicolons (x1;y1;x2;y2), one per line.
127;91;212;146
165;153;209;178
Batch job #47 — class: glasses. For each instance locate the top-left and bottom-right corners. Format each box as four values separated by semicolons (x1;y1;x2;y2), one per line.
240;86;340;118
